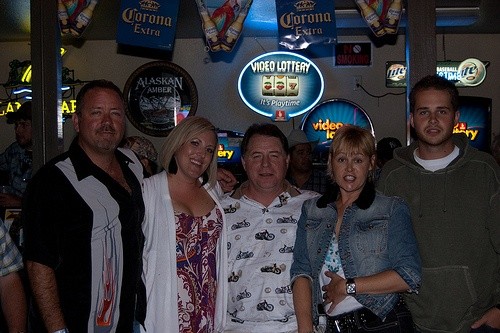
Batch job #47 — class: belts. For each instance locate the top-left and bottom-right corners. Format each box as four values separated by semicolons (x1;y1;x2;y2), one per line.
330;294;408;332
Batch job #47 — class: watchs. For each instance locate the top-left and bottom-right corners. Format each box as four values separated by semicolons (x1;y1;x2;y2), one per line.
346;278;356;296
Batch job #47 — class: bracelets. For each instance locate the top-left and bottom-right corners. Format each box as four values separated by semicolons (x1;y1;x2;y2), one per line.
54;327;69;333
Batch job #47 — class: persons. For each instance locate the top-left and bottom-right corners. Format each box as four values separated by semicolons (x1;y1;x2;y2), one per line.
0;76;499;333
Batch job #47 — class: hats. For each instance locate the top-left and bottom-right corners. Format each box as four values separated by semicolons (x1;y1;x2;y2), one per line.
7;101;32;120
286;129;321;152
123;136;158;162
377;137;403;159
